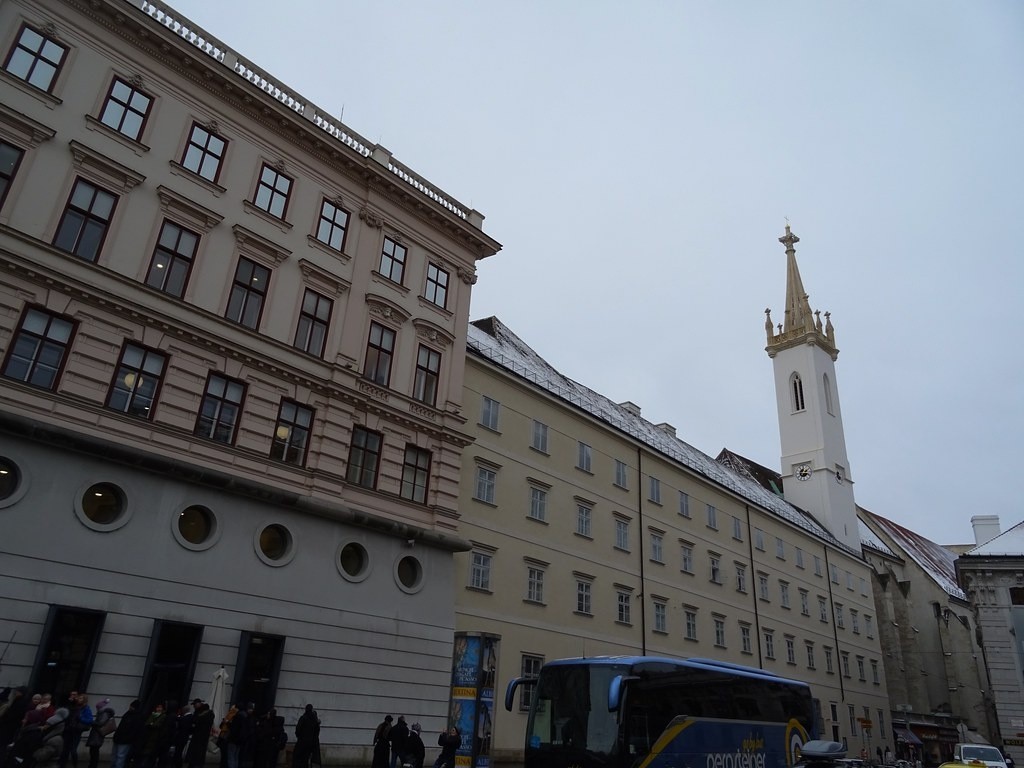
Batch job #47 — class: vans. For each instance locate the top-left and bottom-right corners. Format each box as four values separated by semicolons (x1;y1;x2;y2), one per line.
953;743;1012;768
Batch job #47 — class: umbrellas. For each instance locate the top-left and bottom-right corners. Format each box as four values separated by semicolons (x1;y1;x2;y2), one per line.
207;665;229;728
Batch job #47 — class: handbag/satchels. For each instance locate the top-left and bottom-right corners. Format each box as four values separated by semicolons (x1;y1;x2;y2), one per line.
96;717;117;736
275;731;288;750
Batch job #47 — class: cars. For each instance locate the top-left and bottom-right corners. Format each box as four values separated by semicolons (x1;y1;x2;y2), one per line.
938;762;988;768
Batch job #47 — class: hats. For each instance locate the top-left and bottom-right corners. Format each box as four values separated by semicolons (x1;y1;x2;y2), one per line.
454;726;461;735
96;697;111;709
46;707;69;725
130;699;140;710
191;699;202;705
412;722;421;730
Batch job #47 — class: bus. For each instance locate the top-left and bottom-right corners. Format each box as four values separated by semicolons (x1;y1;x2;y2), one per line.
504;654;823;768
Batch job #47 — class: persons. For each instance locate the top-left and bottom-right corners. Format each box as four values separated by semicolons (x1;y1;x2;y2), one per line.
434;727;462;768
861;749;868;760
0;680;322;768
1005;754;1015;768
875;745;891;764
372;715;425;768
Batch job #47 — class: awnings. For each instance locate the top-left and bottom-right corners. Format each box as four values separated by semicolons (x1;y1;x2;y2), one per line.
894;729;922;744
965;731;990;744
